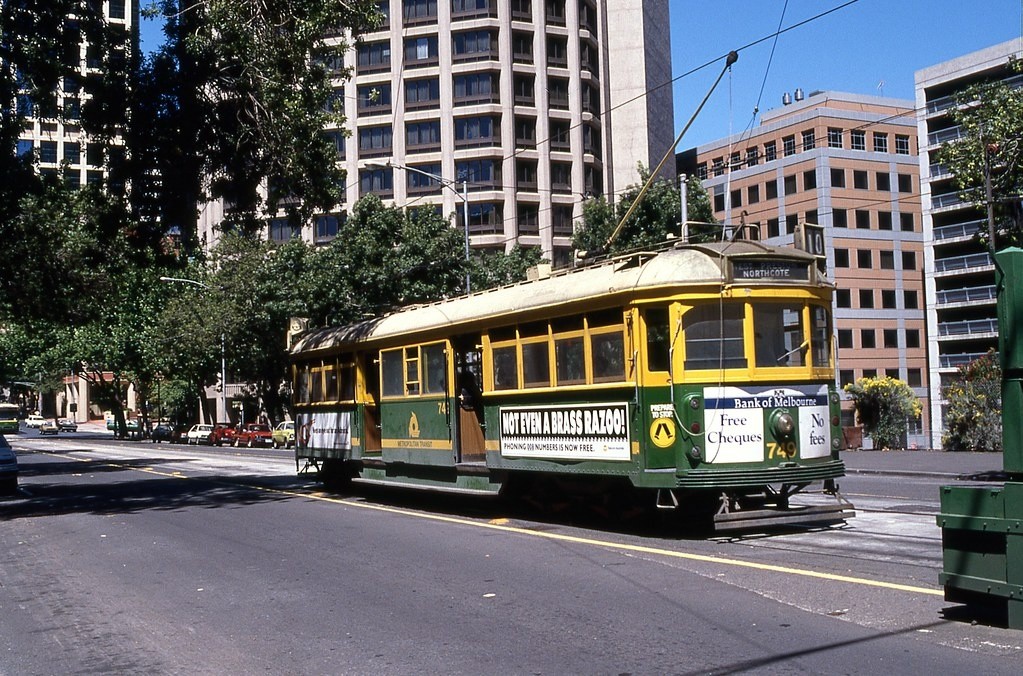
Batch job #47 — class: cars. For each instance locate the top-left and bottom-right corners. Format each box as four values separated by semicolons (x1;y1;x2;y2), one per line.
39;422;58;435
272;420;294;449
152;424;176;444
56;418;78;432
207;422;238;447
170;424;193;444
0;431;18;484
187;424;214;445
232;422;274;448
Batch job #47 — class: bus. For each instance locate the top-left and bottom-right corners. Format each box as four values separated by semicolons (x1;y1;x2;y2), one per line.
291;50;855;536
0;402;21;434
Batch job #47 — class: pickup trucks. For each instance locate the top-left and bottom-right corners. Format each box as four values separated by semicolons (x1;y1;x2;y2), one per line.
24;415;48;429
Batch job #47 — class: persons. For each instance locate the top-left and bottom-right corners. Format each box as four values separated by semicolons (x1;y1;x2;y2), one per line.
722;313;778;368
911;442;917;449
137;411;144;434
882;445;891;452
846;442;856;451
146;413;153;433
459;372;482;407
497;348;541;388
106;417;111;425
785;349;790;362
648;330;717;371
561;339;585;380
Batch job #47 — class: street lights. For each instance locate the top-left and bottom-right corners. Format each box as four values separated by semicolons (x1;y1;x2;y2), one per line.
158;276;228;430
362;161;470;293
